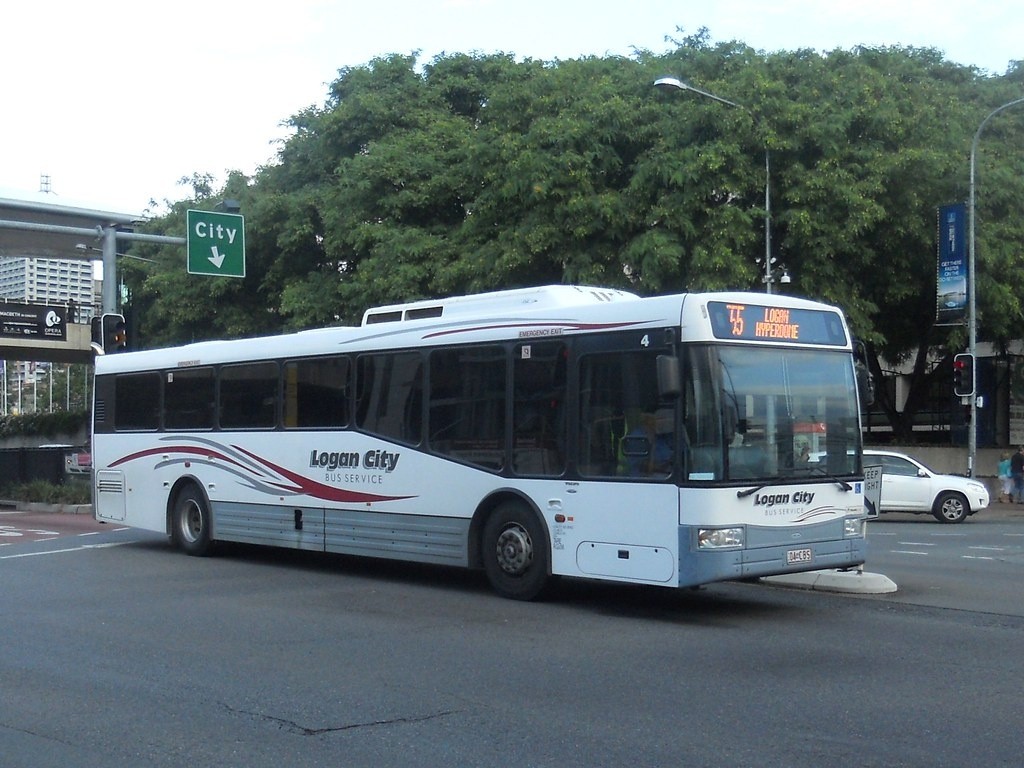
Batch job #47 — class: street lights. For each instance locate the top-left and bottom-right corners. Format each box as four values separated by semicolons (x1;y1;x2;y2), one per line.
651;76;772;289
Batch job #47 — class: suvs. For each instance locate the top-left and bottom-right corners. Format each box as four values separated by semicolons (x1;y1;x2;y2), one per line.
805;448;991;522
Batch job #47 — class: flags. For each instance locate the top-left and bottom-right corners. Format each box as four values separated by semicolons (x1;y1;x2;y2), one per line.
931;202;969;327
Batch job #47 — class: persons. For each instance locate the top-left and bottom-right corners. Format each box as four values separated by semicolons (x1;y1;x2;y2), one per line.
995;449;1013;503
607;366;694;478
66;298;80;323
800;441;811;463
1008;444;1024;504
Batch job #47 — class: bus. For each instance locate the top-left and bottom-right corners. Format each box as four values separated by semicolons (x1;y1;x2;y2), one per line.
87;282;861;599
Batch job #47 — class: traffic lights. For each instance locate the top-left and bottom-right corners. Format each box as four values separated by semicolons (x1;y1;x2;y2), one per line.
951;352;976;398
101;313;128;353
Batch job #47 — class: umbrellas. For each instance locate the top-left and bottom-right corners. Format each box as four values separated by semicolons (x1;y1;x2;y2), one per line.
792;434;810;448
